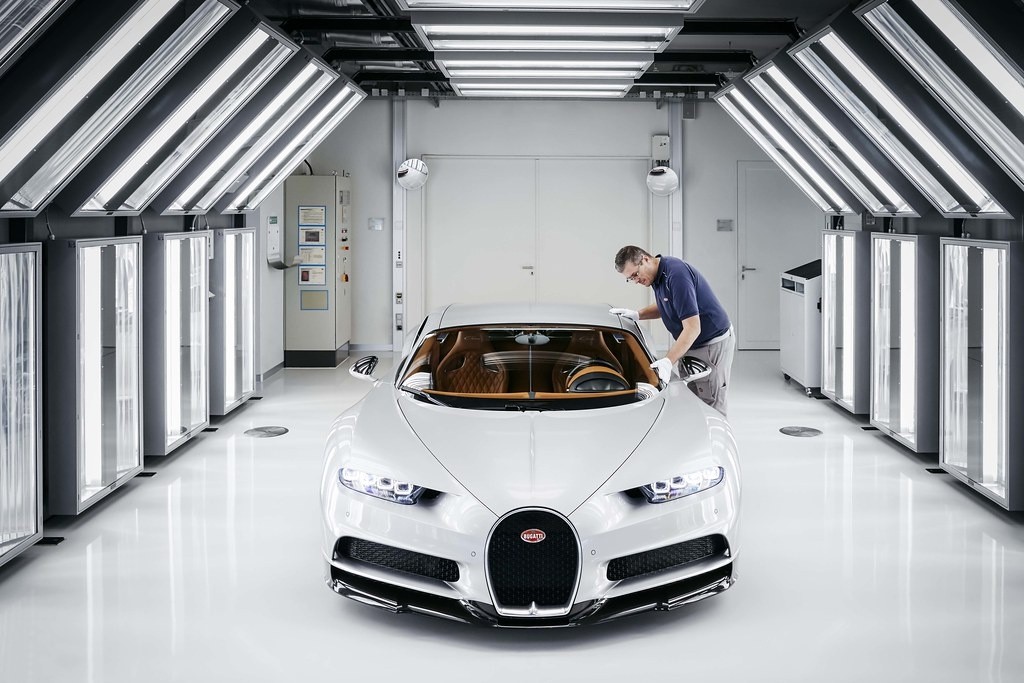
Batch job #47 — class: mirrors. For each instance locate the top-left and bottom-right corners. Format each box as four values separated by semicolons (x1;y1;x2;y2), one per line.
645;166;679;196
397;158;429;191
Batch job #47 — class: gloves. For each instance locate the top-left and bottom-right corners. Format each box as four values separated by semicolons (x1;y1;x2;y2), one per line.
650;357;673;384
610;307;639;321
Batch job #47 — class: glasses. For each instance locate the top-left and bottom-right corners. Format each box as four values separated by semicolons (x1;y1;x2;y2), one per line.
626;260;643;283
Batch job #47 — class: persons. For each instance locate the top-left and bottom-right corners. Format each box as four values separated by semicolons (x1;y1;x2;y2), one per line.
608;245;737;417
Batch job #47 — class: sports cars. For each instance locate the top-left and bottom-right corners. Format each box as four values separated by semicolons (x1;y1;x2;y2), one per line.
318;300;741;631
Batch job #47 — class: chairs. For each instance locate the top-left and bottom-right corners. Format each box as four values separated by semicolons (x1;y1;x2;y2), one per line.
552;329;624;392
435;329;509;393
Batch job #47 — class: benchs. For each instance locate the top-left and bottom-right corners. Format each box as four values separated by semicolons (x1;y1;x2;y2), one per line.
440;333;571;393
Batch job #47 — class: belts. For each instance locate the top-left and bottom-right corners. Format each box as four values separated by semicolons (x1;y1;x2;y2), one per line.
696;324;734;348
